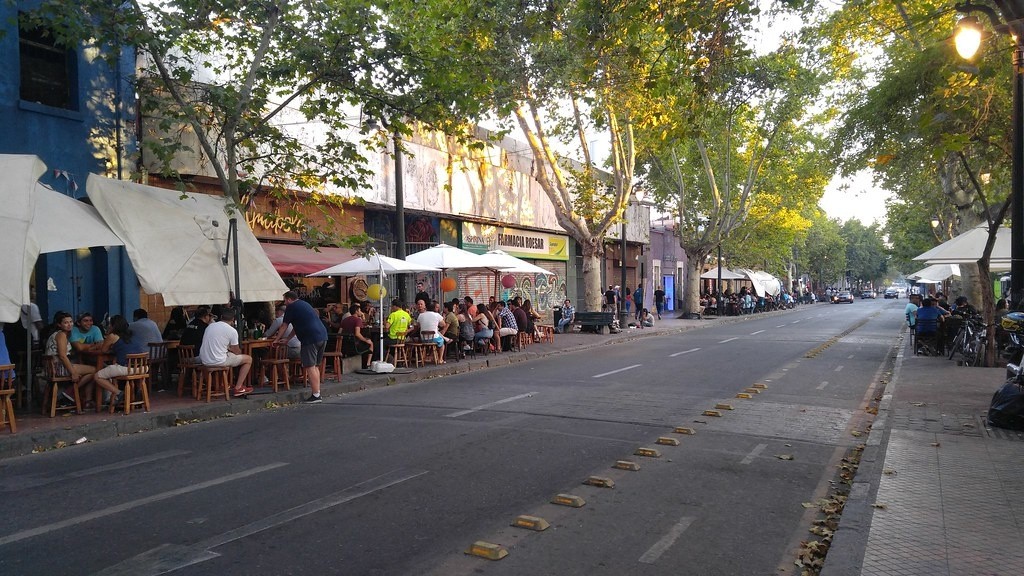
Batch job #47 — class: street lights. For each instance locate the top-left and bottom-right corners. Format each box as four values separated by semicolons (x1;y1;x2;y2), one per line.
363;107;410;312
618;180;645;329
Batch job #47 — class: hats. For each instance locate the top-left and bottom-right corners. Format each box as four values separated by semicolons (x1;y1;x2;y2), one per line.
76;312;91;321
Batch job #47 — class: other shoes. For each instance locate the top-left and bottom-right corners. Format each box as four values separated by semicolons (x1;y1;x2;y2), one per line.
61;389;74;403
257;375;269;384
83;400;96;412
112;390;123;406
463;345;472;350
438;359;447;364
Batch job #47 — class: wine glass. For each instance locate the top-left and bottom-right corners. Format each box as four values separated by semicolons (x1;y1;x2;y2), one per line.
103;321;110;338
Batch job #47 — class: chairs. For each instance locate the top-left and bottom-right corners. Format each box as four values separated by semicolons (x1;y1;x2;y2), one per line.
0;315;557;434
905;314;962;358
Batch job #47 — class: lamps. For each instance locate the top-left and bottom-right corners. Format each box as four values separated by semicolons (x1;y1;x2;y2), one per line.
977;167;994;186
929;214;940;228
945;15;992;59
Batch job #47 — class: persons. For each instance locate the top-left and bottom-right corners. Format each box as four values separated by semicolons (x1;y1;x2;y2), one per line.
640;308;654;327
633;284;644;320
557;299;575;333
0;328;17;401
20;284;329;414
906;291;1010;357
322;284;542;369
653;285;667;319
776;287;856;307
601;284;633;317
699;287;774;316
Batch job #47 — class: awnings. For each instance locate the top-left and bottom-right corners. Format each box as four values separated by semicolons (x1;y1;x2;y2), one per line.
0;154;49;416
85;172;290;350
260;242;366;276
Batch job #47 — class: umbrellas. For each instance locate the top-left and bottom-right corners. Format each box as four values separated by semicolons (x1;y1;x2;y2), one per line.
306;252;442;360
700;263;780;297
446;250;556;300
904;219;1012;296
406;240;516;306
32;181;122;255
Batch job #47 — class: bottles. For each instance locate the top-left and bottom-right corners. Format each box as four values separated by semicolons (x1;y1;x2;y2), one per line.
243;321;248;341
254;323;258;340
259;323;263;338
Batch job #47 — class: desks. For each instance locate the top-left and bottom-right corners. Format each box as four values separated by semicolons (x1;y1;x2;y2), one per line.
88;350;113;413
165;340;180;349
239;339;274;388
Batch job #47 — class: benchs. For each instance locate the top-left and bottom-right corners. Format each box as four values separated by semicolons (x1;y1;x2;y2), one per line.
567;312;615;335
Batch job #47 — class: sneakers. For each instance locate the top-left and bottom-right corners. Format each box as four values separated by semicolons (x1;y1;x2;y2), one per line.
233;386;253;397
304;393;323;404
221;383;236;391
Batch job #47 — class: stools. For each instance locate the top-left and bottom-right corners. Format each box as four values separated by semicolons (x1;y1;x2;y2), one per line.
196;365;231;404
406;342;425;368
514;332;526;352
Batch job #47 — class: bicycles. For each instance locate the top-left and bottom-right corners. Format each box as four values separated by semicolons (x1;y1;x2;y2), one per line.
948;309;1001;367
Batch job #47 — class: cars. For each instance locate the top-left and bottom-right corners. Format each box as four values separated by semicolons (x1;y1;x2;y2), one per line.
884;289;899;299
861;290;876;299
834;290;853;304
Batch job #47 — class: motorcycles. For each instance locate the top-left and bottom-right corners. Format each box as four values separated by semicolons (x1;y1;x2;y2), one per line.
1001;312;1024;386
